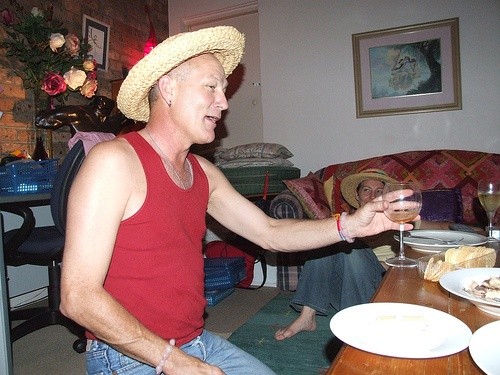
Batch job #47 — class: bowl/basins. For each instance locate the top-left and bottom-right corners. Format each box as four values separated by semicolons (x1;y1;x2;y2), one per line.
416;248;497;282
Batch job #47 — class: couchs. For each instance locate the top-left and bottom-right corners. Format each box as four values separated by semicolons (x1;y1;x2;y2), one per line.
269;149;500;291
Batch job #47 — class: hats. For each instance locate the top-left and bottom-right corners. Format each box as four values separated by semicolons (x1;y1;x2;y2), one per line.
116;26;246;123
340;168;401;209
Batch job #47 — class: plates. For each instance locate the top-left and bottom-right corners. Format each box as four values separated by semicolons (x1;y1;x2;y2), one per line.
394;230;489;253
329;302;472;358
438;267;500;316
469;321;500;375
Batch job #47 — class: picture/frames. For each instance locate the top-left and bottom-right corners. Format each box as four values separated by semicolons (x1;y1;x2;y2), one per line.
351;16;462;119
81;14;111;73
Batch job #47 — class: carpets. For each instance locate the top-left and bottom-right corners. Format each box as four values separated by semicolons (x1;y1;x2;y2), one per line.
228;288;342;375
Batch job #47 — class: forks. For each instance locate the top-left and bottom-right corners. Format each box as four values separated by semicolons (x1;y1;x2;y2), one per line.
410;236;464;244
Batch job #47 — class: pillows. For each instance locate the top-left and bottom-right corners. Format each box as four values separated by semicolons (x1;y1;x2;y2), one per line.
416;188;465;223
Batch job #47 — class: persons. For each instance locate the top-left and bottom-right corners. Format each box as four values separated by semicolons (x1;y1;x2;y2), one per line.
59;25;421;375
274;169;421;339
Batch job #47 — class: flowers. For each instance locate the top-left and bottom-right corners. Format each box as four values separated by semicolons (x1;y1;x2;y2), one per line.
0;0;98;99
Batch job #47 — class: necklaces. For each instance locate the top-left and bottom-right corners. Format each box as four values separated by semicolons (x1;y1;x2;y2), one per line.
145;127;185;189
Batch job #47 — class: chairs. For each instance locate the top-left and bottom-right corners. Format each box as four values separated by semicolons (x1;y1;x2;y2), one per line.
2;133;118;353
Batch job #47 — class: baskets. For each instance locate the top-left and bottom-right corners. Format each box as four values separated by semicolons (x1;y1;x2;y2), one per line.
203;256;247;306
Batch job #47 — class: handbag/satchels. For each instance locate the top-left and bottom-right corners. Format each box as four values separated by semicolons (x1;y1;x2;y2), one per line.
206;238;267;289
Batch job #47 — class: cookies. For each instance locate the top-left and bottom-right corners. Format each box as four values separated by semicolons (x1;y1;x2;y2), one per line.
423;245;496;282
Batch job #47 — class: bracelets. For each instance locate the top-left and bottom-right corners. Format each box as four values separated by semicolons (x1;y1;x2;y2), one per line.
155;339;175;375
340;212;355;243
335;213;345;241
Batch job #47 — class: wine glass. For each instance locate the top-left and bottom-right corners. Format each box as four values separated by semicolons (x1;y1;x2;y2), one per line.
383;182;423;267
478;178;500;242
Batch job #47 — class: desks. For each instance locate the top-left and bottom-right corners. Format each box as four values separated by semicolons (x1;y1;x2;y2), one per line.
0;187;52;375
326;234;498;375
